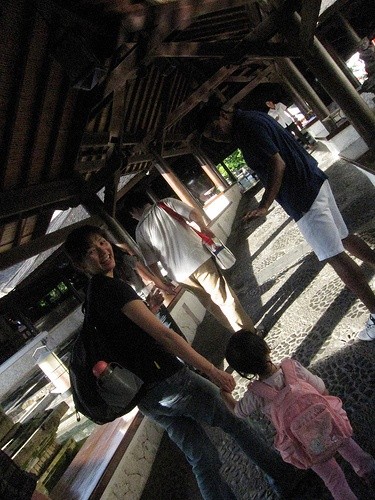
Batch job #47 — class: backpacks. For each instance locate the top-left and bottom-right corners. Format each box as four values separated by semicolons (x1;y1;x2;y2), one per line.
247;359;353;469
67;274;149;425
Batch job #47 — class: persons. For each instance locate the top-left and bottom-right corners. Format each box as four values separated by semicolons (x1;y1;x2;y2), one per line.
219;329;375;500
196;106;375;340
266;99;314;148
356;37;375;76
65;226;309;500
109;242;201;373
124;195;268;339
0;448;50;500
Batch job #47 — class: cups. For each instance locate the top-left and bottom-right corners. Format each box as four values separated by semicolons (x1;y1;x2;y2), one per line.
92;361;114;381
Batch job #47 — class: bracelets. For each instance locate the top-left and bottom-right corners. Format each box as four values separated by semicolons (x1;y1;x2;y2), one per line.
207;364;213;375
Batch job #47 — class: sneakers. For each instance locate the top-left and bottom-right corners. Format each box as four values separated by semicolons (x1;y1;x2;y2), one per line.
359;314;375;341
307;144;313;149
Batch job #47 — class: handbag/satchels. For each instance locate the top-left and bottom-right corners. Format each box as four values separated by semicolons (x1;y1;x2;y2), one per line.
203;238;236;270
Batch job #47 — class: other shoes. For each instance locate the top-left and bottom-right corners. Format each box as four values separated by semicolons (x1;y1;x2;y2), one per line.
256;324;265;337
